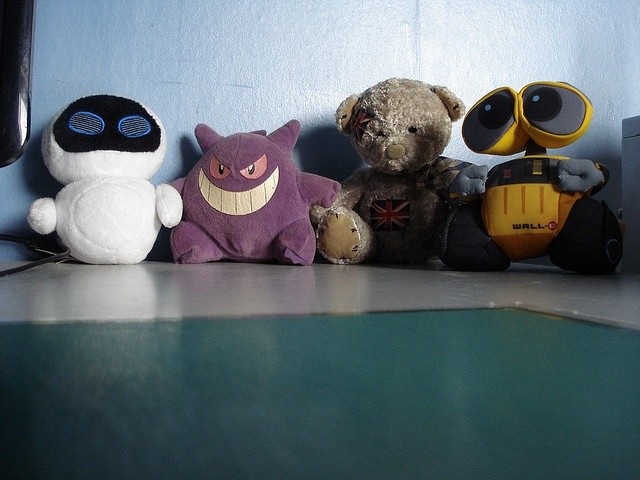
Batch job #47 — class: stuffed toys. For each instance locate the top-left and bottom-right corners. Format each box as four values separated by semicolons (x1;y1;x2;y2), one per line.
434;81;624;277
308;76;475;265
25;93;184;265
166;119;342;267
